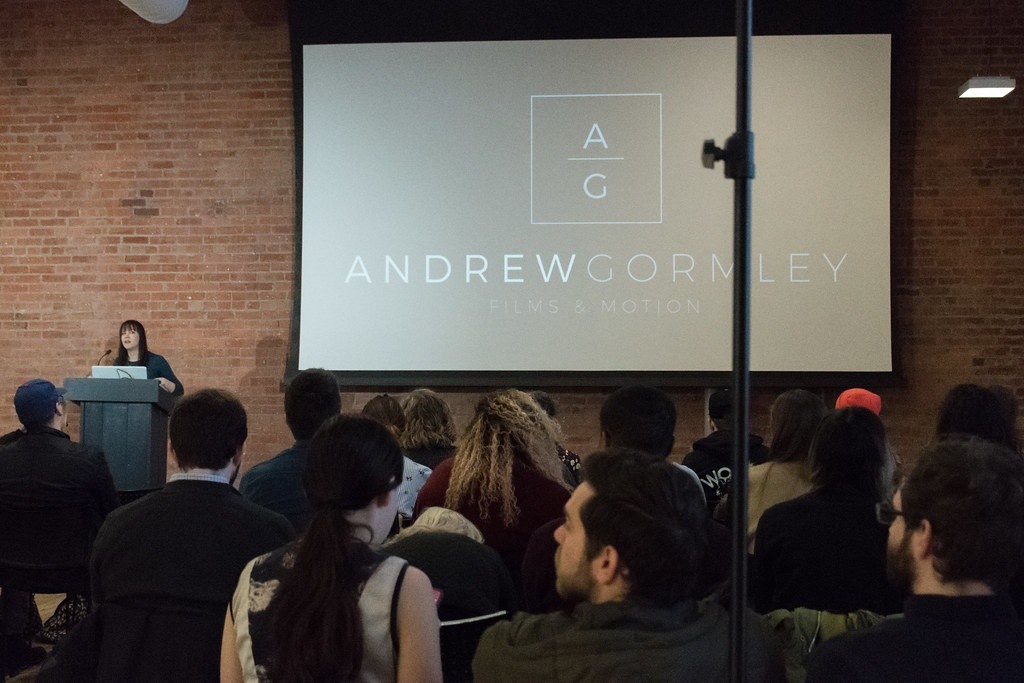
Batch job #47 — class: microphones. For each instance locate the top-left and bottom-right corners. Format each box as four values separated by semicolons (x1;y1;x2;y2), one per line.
98;349;111;366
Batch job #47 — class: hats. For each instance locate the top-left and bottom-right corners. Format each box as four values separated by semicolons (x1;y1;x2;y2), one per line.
14;379;68;423
709;389;734;418
836;389;881;415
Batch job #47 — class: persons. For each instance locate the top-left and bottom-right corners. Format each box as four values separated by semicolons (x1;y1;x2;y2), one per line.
239;369;343;533
682;389;771;514
805;432;1024;683
471;450;787;683
361;394;433;517
114;320;185;396
90;389;297;683
937;383;1015;446
522;384;677;613
398;388;459;470
220;410;443;683
412;388;575;579
0;379;120;564
531;390;584;485
752;406;904;614
748;389;829;555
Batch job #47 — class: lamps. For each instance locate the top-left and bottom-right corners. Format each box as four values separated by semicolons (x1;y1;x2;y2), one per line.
957;0;1019;99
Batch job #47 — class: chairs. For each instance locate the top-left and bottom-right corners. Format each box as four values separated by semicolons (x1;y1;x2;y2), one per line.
93;595;226;683
0;450;111;683
520;517;564;582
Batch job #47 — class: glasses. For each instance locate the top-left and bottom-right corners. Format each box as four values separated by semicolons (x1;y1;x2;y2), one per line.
872;501;957;525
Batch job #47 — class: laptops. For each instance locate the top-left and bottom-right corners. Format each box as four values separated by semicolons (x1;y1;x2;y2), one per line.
91;366;147;379
